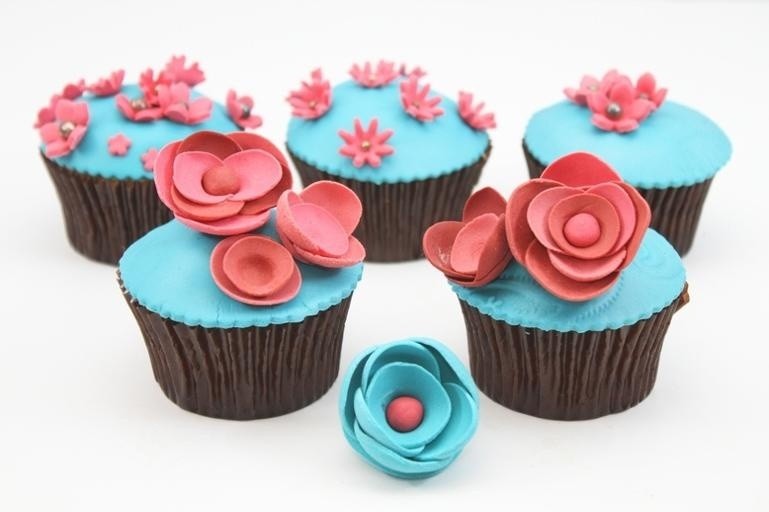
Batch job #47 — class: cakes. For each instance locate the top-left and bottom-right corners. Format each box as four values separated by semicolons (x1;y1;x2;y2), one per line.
286;58;491;262
420;151;690;418
116;130;365;421
33;53;263;265
522;67;731;258
